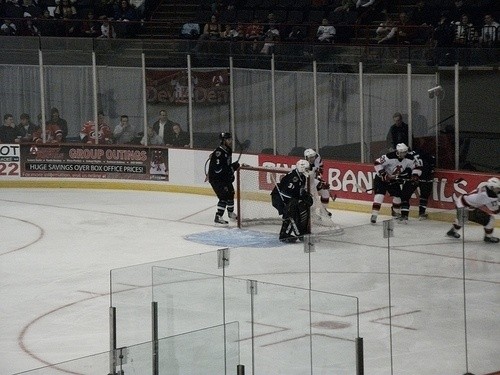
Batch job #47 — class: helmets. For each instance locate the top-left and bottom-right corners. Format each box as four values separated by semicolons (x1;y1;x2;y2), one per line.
304;149;316;164
486;177;500;195
220;131;232;139
295;159;310;177
396;143;409;157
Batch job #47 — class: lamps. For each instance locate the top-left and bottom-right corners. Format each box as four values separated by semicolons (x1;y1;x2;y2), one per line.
428;85;443;98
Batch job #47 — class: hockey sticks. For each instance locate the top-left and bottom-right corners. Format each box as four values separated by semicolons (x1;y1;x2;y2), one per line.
403;177;464;184
270;173;321;243
328;189;337;202
355;184;373;193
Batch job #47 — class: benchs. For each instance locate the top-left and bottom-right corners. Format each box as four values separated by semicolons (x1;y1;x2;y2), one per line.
142;0;203;38
347;0;420;45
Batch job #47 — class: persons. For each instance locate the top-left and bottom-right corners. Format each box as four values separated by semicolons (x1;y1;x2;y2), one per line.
0;0;500;67
1;107;190;149
208;132;240;224
271;148;331;244
447;177;500;243
145;71;225;105
370;112;433;224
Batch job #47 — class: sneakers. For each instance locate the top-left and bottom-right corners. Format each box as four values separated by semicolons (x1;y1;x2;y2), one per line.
483;235;499;243
370;215;378;225
446;231;461;238
279;233;304;243
397;213;429;224
325;208;332;217
391;213;401;217
214;212;238;224
